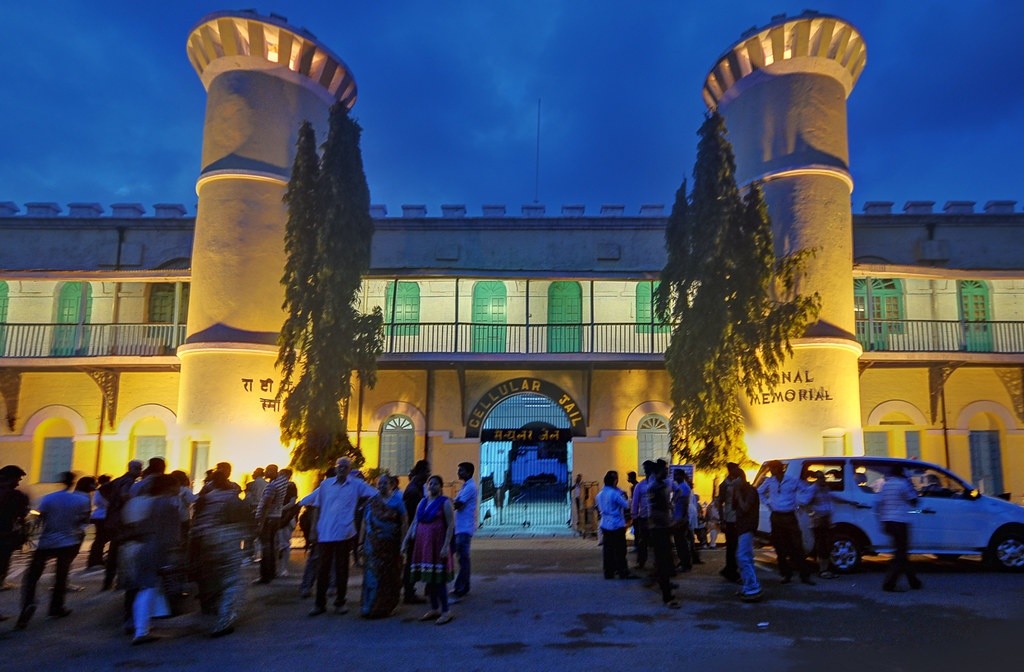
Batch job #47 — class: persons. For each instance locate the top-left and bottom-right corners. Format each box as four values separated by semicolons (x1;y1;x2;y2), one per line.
400;476;454;626
13;471;96;630
402;459;431;606
693;492;723;549
98;461;142;591
244;465;298;585
283;456;408;620
873;465;924;593
595;459;707;605
0;465;32;623
452;462;478;603
86;475;111;568
717;461;833;596
114;458;257;646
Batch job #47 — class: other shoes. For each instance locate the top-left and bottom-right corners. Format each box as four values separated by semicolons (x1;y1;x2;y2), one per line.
909;580;922;590
303;590;312;599
207;625;235;639
252;577;271;585
819;571;840;580
781;574;792;584
670;560;705;588
446;588;466;606
363;614;375;620
881;583;900;592
308;606;327;617
334;604;348;614
403;592;428;605
418;608;442;622
130;633;161;646
667;600;681;609
636;564;646;572
719;569;740;582
623;572;643;580
799;577;817;585
47;607;74;619
605;574;616;580
16;598;39;631
436;608;456;623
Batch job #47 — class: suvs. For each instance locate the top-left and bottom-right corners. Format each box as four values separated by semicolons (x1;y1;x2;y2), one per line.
747;455;1024;578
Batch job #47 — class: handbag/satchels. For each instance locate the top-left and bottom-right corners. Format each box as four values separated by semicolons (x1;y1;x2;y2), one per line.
595;520;605;547
